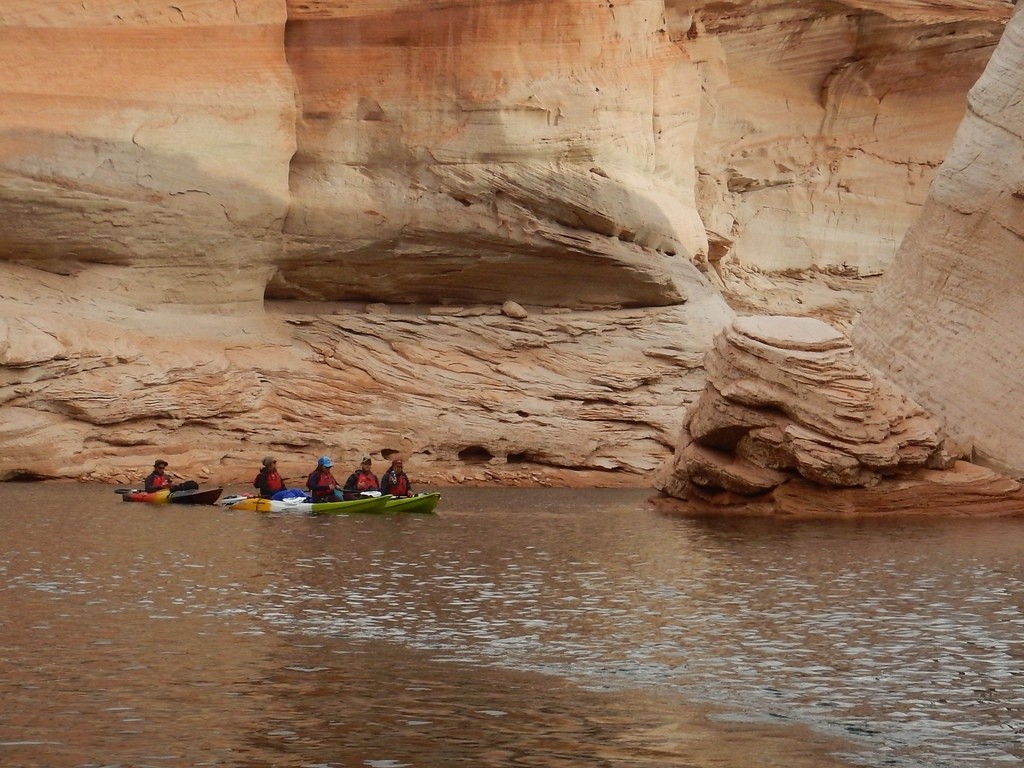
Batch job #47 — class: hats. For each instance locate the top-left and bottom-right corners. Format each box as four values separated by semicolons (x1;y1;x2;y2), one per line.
392;460;402;465
263;456;274;466
318;457;334;467
362;458;371;464
155;459;168;466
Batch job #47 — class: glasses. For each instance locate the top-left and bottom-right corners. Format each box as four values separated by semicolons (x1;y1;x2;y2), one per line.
158;467;165;469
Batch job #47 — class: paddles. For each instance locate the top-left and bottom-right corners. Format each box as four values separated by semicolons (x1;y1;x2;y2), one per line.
114;488;145;495
221;493;261;508
338;487;382;498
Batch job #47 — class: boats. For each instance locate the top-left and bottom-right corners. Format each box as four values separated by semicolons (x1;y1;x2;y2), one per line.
229;494;391;515
122;487;223;505
386;492;442;513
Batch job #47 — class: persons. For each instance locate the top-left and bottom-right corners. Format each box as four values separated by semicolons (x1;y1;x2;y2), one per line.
145;459;173;493
343;458;383;501
381;460;414;497
308;456;342;502
253;456;314;503
